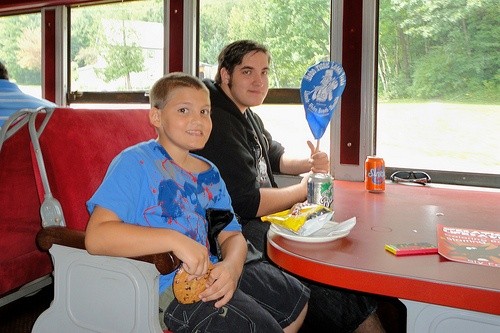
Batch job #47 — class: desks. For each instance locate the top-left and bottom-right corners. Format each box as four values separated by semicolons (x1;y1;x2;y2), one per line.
267;176;500;318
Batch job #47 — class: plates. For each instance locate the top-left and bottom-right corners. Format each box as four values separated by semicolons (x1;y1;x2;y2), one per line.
270;221;351;243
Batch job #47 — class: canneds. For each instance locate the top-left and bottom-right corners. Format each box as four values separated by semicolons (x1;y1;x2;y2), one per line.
306;172;334;219
365;156;385;193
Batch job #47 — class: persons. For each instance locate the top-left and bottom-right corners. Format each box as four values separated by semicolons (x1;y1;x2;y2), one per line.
84;72;311;333
0;60;58;131
189;39;387;333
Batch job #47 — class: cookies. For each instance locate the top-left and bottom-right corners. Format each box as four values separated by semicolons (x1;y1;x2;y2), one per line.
174;261;216;304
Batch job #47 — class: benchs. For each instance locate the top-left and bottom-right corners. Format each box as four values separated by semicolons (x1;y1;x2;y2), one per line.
29;105;182;333
0;106;53;311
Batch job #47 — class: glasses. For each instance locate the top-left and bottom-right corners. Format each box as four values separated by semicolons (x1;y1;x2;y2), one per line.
389;171;432;185
